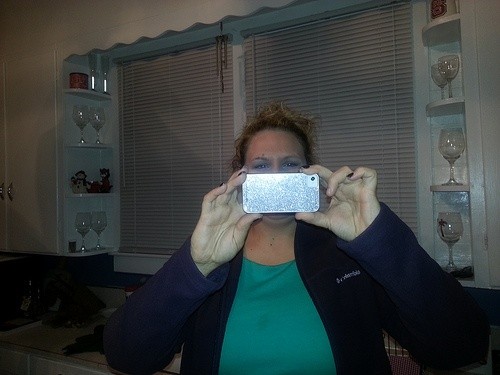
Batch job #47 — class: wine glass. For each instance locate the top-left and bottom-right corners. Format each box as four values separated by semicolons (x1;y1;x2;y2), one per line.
438;128;465;185
102;52;111;93
75;211;92;254
90;53;98;92
437;213;464;272
430;64;448;100
439;55;458;97
73;105;90;144
93;210;108;251
91;106;104;144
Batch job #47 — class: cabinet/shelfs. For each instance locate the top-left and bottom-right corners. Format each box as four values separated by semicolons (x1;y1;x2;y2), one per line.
0;50;58;255
57;54;121;256
412;0;489;289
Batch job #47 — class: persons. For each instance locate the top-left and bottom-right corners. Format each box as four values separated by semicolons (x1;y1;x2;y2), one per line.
102;101;491;375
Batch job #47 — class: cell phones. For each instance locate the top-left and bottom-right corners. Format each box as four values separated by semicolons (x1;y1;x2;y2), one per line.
242;173;320;214
